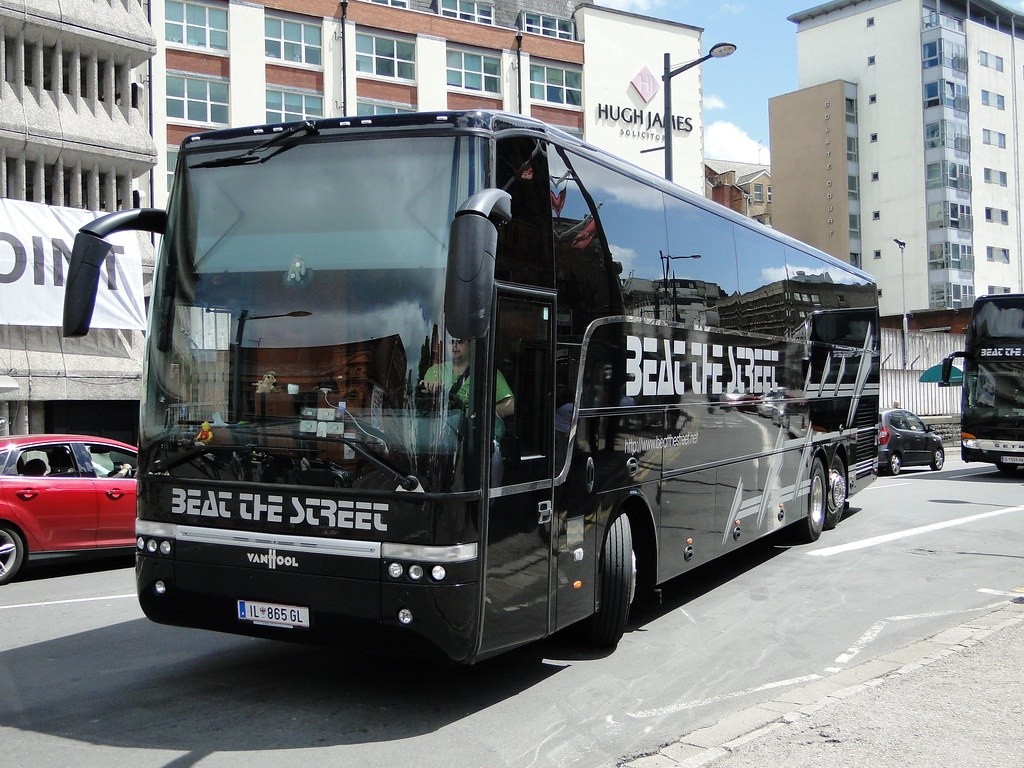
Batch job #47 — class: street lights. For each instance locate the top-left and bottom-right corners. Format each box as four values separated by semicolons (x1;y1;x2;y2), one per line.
894;239;908;369
659;250;701;322
662;42;737;183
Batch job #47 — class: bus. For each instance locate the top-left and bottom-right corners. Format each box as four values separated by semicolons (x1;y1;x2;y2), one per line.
62;108;881;665
791;306;880;384
942;293;1024;470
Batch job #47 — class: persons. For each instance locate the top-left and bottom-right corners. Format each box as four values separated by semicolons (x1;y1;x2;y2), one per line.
422;335;516;506
87;452;132;478
556;365;624;450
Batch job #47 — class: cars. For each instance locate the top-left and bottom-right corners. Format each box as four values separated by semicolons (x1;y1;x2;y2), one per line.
757;387;788;427
0;434;139;585
878;408;945;476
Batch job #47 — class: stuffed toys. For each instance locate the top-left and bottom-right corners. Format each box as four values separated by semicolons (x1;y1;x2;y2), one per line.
256;371;281;393
